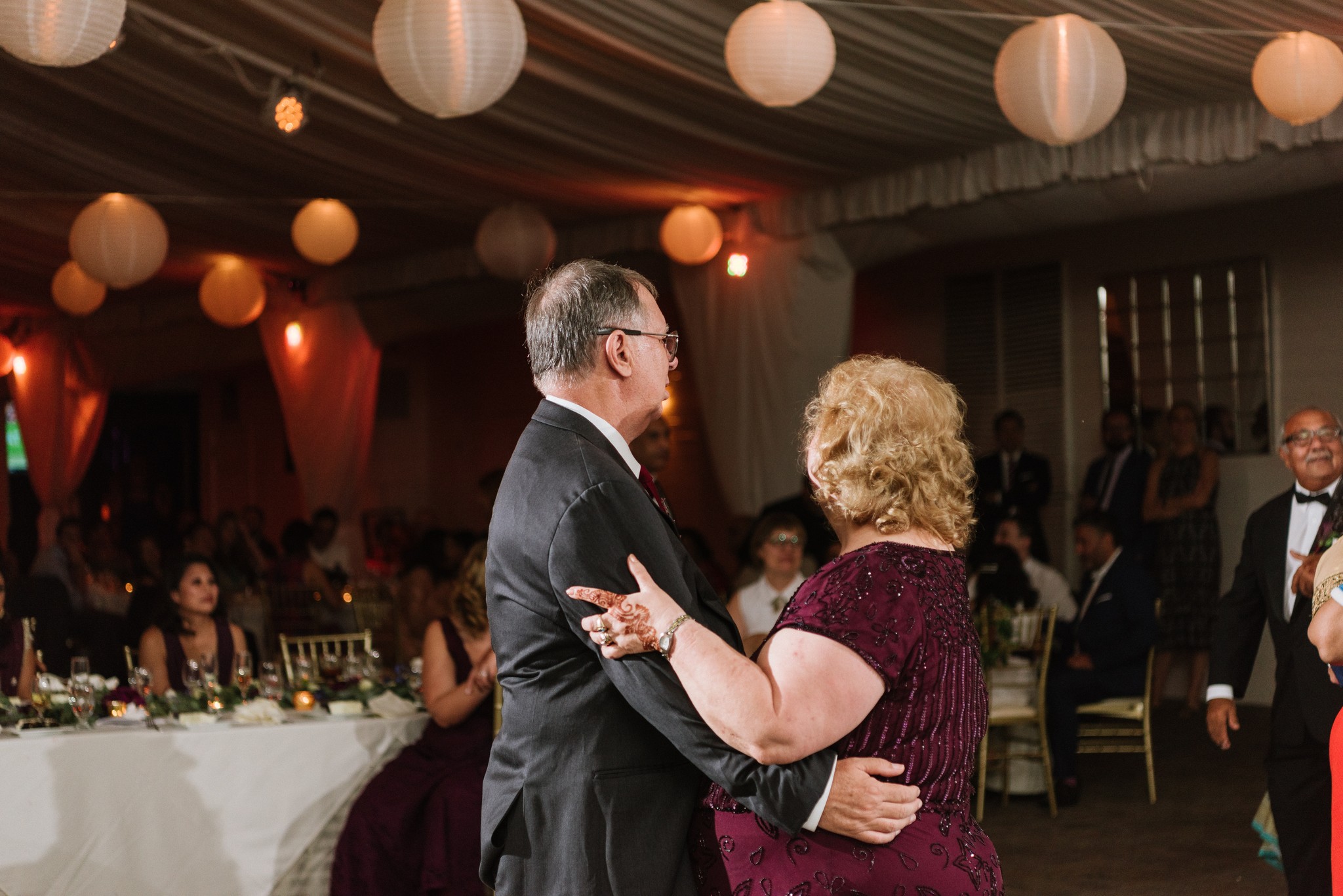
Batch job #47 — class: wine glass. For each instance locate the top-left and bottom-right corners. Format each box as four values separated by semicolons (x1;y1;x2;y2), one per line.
65;649;427;729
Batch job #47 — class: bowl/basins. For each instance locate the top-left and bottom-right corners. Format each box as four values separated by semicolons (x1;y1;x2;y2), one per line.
328;700;363;717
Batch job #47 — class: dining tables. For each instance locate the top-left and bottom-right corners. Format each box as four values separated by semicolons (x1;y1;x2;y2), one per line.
1;681;446;896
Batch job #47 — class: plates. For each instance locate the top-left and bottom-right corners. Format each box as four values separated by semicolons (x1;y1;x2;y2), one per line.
92;717;147;731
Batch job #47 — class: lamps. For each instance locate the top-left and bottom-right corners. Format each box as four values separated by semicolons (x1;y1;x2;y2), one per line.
256;70;311;136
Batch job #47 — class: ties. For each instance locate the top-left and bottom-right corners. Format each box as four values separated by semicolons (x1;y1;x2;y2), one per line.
639;461;676;525
1097;459;1115;505
1008;453;1015;485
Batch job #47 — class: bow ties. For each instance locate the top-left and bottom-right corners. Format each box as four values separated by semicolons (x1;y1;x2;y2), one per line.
1294;491;1330;506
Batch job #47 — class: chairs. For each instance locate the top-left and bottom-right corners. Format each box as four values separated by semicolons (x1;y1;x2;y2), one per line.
1070;596;1166;806
271;629;380;690
969;598;1059;822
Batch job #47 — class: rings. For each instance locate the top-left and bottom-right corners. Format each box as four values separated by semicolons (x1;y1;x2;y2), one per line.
604;632;613;645
597;614;607;633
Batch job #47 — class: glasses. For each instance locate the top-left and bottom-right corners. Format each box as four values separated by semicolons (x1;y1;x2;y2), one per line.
596;327;679;363
1284;427;1342;448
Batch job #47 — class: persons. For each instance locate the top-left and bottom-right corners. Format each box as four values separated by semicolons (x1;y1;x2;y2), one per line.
568;356;1005;896
632;410;838;658
967;401;1218;810
1206;408;1343;896
4;504;497;896
481;258;923;896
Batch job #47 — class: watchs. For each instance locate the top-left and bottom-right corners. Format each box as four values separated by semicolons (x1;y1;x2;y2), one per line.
658;614;694;660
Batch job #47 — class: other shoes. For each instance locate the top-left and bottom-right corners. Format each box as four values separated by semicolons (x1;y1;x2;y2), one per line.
1041;782;1076;807
1179;705;1196;719
